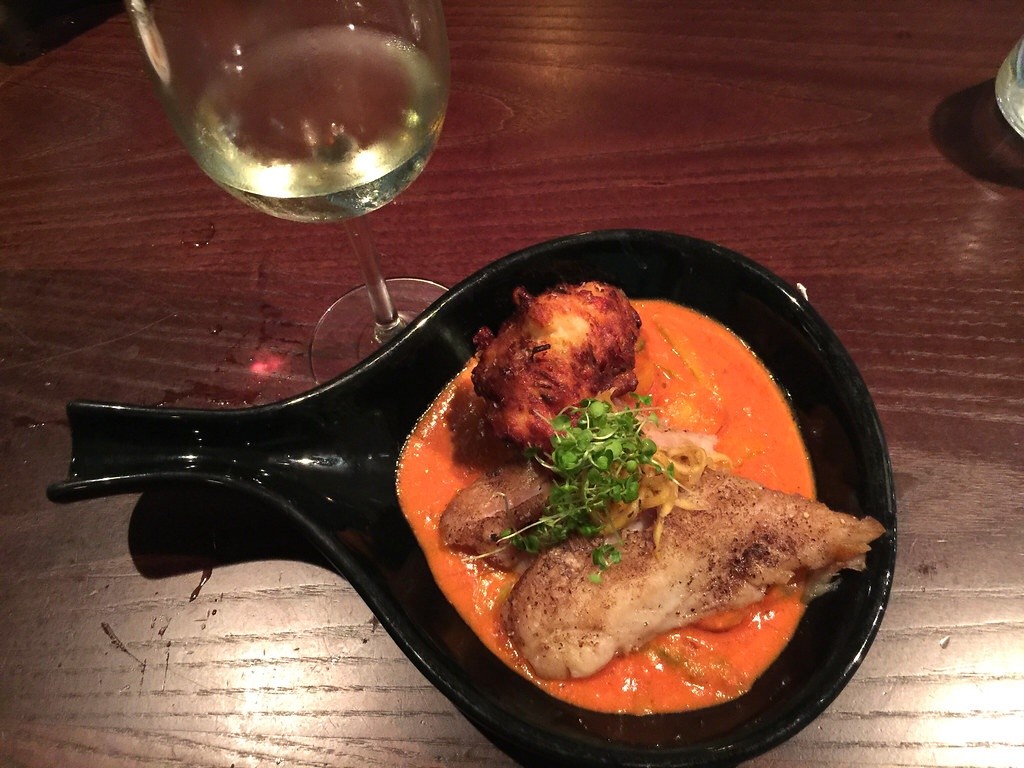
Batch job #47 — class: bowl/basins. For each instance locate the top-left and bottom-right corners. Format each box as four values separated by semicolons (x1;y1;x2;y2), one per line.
38;227;900;768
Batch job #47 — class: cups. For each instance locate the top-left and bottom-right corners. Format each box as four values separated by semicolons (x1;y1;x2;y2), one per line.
994;34;1024;139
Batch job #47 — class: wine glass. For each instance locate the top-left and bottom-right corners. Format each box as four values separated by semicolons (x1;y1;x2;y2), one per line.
122;0;452;389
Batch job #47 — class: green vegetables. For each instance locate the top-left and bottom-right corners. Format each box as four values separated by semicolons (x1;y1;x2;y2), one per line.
457;392;696;583
